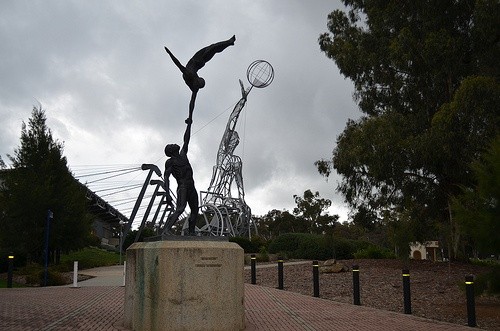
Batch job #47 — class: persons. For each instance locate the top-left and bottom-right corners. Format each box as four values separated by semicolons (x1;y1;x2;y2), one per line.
163;34;236;122
201;77;253;240
162;120;199;237
